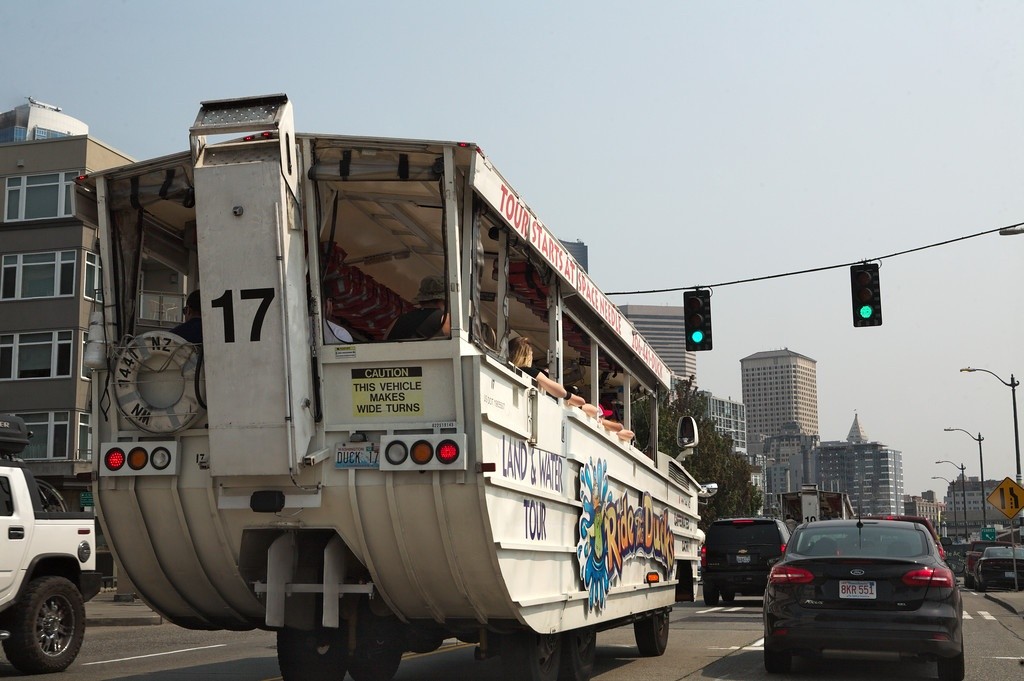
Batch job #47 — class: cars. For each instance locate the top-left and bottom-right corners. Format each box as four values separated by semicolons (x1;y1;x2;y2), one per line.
973;546;1024;591
762;514;966;681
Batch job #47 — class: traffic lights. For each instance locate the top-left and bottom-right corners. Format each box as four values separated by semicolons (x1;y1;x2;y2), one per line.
851;262;881;327
682;288;713;353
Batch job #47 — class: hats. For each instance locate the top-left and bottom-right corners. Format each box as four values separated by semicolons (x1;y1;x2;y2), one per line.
413;275;445;301
598;404;613;417
569;385;584;394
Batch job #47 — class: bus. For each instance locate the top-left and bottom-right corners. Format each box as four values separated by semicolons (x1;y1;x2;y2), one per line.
69;102;703;681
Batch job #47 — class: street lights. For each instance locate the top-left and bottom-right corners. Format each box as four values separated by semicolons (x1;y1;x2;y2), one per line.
936;459;969;543
933;476;958;541
959;363;1022;529
943;427;989;542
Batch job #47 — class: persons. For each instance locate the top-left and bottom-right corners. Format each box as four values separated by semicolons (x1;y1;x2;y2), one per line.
508;337;567;399
563;386;636;441
167;290;202;344
378;275;451;340
321;282;352;344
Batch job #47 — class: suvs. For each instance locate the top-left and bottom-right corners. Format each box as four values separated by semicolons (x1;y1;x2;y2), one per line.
4;413;102;674
964;540;1017;587
700;516;789;603
